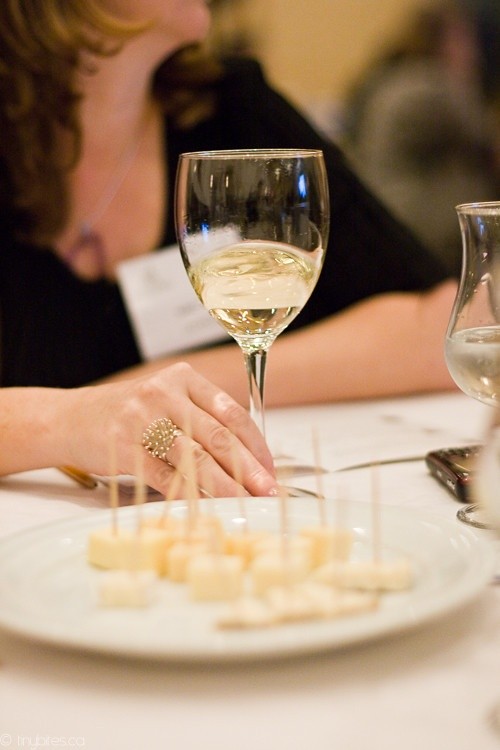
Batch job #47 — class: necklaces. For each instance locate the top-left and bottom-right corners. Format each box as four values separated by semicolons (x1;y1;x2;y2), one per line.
61;142;144;277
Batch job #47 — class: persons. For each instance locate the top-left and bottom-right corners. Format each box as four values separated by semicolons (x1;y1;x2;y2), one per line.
0;1;500;499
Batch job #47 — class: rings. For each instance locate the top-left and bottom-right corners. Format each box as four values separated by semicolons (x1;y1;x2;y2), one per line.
142;418;186;462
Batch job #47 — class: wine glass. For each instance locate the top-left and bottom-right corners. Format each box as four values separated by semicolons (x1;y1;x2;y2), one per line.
172;148;330;498
442;200;500;531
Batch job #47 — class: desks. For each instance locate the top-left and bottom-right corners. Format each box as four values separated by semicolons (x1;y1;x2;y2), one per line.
0;386;500;749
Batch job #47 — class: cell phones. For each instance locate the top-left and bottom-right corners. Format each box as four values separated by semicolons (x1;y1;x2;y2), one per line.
425;444;486;504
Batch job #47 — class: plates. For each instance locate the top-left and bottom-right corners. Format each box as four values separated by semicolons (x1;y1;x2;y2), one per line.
0;497;499;665
